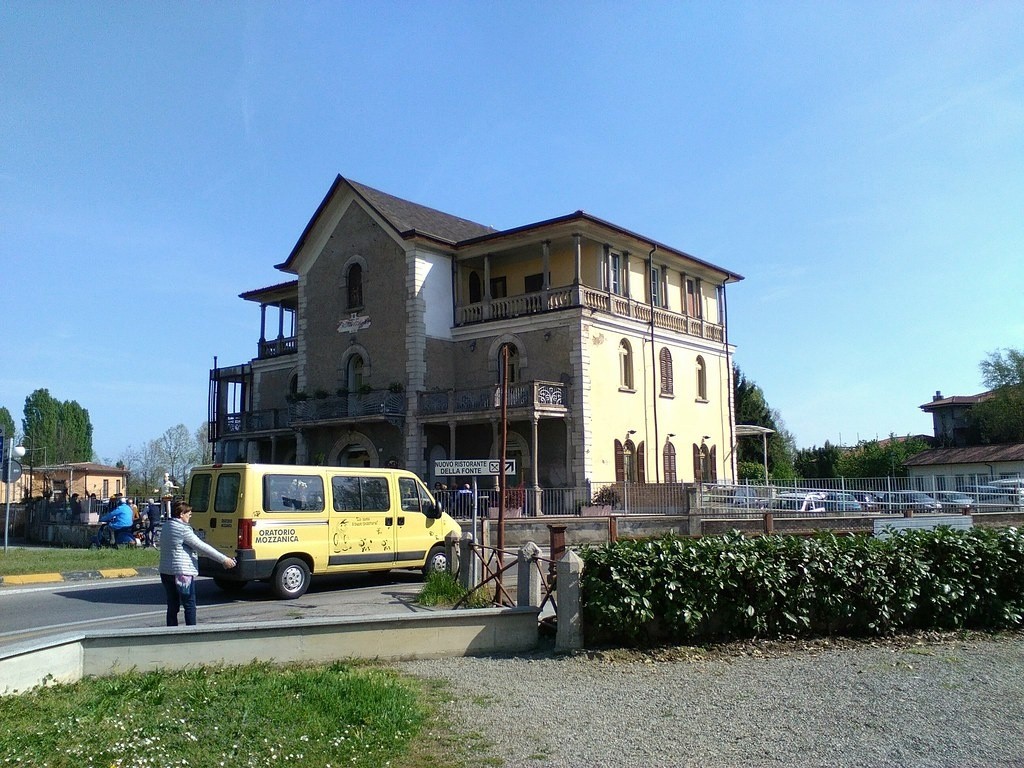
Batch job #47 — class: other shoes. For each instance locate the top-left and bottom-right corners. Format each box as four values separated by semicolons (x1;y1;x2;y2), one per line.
107;539;115;544
144;542;151;547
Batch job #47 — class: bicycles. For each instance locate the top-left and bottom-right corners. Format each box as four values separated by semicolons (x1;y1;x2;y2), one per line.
134;519;164;552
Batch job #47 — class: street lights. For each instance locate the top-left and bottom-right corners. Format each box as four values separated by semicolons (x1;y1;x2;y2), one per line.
13;435;33;545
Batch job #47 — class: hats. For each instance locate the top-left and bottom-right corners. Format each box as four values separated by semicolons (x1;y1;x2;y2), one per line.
147;499;154;504
109;494;115;498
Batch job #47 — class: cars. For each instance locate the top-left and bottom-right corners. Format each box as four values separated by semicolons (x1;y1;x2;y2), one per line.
698;476;1023;513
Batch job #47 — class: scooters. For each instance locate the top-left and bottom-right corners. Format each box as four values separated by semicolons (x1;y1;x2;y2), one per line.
87;518;138;552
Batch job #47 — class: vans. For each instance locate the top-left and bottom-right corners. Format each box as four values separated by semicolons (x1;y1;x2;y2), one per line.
179;462;464;599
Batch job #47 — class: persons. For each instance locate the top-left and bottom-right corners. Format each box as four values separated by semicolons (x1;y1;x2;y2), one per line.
159;502;236;627
71;490;161;548
159;472;174;520
430;482;473;520
